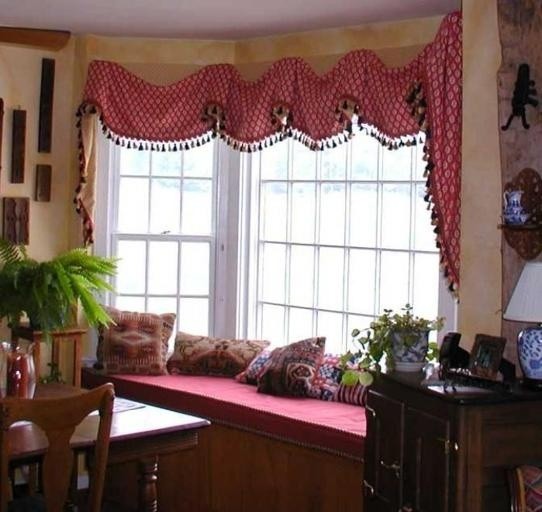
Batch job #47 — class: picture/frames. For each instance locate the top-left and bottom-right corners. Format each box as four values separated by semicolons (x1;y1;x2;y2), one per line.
468;334;507;378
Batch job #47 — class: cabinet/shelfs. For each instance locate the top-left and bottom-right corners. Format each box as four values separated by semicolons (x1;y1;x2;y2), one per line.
366;382;542;510
7;321;88;388
111;378;365;512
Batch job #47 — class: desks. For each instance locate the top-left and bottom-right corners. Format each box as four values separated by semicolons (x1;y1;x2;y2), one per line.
10;399;211;511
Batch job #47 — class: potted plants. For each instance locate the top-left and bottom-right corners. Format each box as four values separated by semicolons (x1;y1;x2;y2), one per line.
339;305;444;387
1;236;117;397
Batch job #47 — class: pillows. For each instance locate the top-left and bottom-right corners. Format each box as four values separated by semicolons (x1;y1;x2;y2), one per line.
167;331;271;377
257;336;327;397
236;351;369;405
93;304;178;376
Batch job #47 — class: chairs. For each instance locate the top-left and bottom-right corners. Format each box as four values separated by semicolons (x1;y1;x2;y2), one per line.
1;383;115;512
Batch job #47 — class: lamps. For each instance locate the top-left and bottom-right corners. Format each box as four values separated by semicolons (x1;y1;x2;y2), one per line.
502;263;542;327
